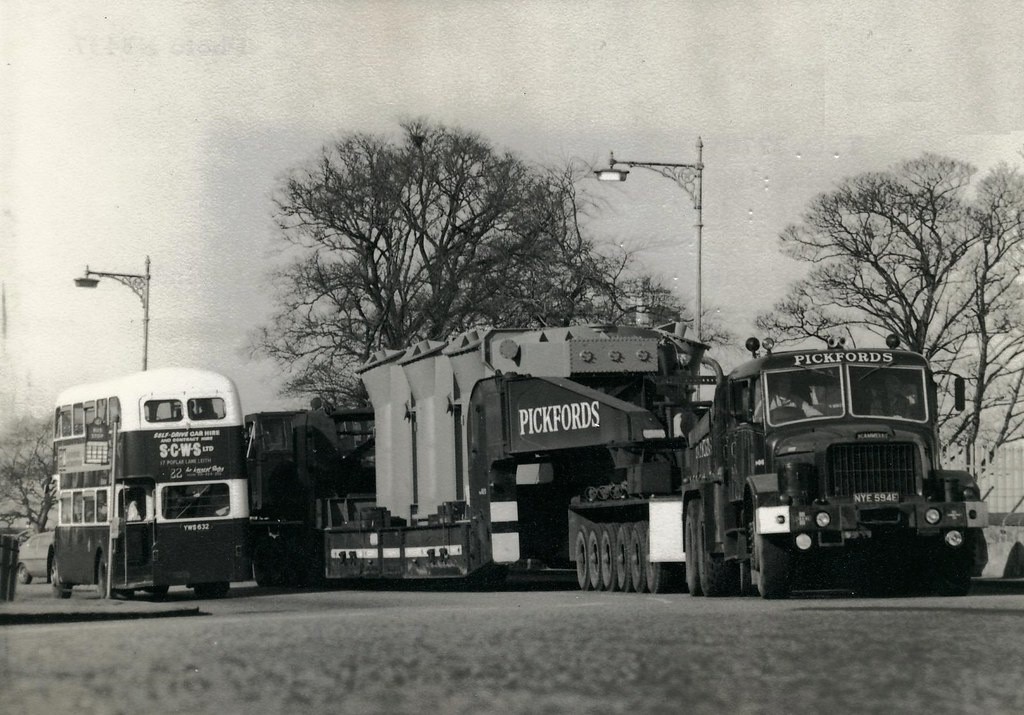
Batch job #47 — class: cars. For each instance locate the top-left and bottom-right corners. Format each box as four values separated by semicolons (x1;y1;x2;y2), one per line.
17;531;57;584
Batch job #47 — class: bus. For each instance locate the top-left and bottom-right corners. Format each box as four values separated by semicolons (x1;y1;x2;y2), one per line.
48;367;254;599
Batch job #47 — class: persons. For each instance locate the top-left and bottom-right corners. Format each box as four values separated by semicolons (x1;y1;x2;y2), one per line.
752;372;824;427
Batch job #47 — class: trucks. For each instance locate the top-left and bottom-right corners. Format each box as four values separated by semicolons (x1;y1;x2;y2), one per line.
685;334;991;599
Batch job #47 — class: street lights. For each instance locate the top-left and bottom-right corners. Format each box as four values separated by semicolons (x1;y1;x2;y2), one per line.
72;254;152;371
593;136;705;339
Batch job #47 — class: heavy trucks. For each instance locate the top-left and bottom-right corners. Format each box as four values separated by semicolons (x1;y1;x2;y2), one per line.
244;321;725;595
